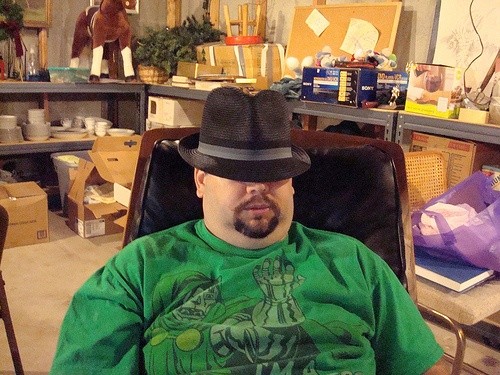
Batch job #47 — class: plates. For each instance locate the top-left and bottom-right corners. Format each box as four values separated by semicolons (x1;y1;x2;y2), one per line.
51;127;87;139
0;109;52;143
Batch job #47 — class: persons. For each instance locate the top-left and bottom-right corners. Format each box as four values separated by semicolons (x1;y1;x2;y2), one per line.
50;86;449;375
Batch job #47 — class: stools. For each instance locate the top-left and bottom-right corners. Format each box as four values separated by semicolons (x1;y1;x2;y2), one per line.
223;4;263;45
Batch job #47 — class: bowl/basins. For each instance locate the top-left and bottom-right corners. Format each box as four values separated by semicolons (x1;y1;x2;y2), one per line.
85;117;135;137
48;66;91;84
60;115;84;128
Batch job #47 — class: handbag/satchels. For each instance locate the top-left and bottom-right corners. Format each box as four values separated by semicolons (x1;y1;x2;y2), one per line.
410;172;500;272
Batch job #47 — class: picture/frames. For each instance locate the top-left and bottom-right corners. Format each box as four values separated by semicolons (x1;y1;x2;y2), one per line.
14;0;52;28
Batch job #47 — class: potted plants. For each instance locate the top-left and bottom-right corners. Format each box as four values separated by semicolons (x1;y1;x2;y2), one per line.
132;14;226;85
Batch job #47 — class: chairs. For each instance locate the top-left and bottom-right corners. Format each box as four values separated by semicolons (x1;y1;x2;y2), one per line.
123;128;465;375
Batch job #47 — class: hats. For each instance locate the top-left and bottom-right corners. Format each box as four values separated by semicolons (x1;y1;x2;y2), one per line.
178;87;311;182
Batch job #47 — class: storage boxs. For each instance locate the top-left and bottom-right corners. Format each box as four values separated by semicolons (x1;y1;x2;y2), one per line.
68;96;207;239
409;132;500;190
0;181;49;249
177;61;222;79
301;63;455;109
47;67;90;83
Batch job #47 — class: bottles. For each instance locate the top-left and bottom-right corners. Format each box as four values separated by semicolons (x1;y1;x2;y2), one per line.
28;48;40;81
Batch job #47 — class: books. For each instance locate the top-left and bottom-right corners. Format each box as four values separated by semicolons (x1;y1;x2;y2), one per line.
414;249;495;292
172;75;192;88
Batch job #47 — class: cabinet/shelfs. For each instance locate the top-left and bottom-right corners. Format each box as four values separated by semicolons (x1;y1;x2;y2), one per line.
0;82;145;207
395;109;500;342
146;83;399;148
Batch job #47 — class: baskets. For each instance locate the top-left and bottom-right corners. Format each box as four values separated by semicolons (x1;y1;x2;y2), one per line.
139;65;169;84
